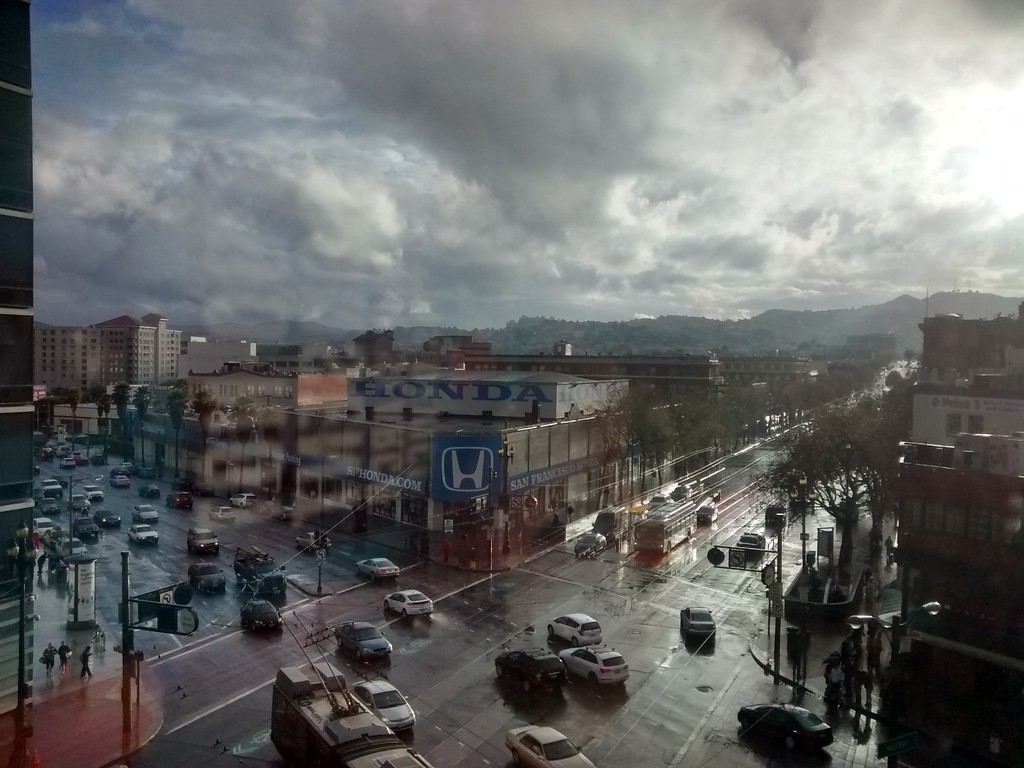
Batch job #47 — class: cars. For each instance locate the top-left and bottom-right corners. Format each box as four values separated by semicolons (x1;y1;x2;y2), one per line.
505;724;596;768
33;445;294;594
296;532;332;553
574;484;721;554
240;557;434;661
736;502;787;557
352;680;416;732
680;607;716;639
495;613;630;693
738;704;834;752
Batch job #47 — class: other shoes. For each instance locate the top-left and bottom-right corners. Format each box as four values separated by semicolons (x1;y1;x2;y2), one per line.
82;676;87;679
90;673;95;677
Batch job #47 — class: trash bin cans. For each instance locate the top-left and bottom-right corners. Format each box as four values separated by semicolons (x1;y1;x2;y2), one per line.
786;626;800;660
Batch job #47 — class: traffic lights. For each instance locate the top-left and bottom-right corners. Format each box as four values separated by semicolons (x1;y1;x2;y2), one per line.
313;531;328;548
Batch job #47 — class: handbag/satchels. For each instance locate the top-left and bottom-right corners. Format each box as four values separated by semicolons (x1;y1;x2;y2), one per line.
65;651;72;658
39;656;47;664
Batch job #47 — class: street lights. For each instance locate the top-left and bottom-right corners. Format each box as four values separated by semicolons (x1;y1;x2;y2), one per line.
885;531;927;713
790;472;818;570
7;519;38;768
499;436;515;553
847;600;941;768
69;474;104;555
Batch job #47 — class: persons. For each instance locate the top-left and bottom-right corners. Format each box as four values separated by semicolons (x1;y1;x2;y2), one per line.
567;506;574;522
885;536;893;548
80;646;93;679
793;631;882;706
603;487;610;500
43;642;57;676
443;538;449;562
37;552;49;575
57;641;72;674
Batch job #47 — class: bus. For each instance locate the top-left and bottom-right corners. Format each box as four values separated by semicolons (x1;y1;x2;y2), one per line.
270;661;433;768
634;501;698;554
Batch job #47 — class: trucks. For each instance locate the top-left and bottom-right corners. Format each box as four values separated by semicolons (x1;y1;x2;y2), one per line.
234;544;287;599
